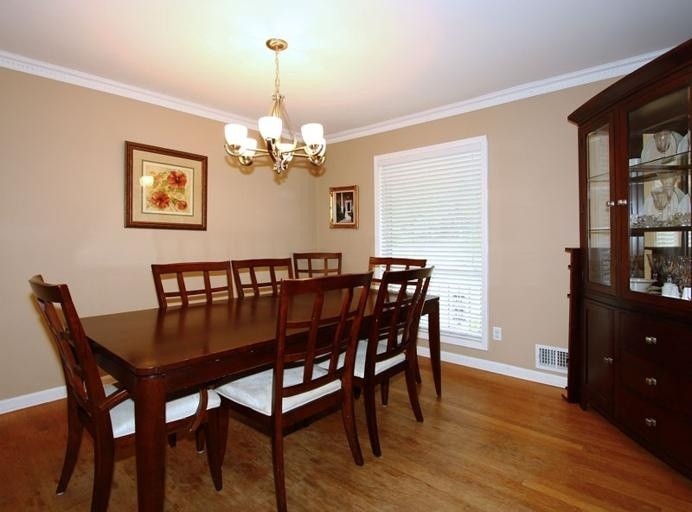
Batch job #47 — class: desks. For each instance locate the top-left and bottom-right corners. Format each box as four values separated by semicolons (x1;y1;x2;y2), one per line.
55;286;442;512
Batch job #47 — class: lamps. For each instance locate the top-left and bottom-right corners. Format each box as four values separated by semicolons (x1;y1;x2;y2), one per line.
223;38;326;175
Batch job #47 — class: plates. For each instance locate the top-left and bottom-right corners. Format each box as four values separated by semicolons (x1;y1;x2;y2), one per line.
640;132;676;166
643;188;678;220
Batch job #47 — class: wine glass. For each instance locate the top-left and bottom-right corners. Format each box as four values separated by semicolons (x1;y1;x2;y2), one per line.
648;186;677;227
650;172;680;227
652;130;672;164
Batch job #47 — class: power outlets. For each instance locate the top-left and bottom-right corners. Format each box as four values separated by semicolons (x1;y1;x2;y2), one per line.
493;327;502;342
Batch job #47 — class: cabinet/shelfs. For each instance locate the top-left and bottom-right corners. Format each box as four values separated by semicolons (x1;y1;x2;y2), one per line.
567;37;692;482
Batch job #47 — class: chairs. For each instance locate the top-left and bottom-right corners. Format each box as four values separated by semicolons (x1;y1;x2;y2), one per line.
232;258;295;298
301;266;434;458
152;260;234;307
366;256;427;407
27;273;230;512
293;252;343;278
215;270;375;510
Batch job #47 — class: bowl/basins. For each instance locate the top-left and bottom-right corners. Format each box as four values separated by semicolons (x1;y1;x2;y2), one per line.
630;276;658;294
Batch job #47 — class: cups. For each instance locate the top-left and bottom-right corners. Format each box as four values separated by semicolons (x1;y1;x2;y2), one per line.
659;281;692;302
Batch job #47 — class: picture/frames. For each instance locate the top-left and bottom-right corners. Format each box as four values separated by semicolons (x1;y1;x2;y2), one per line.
125;140;207;231
328;184;359;229
